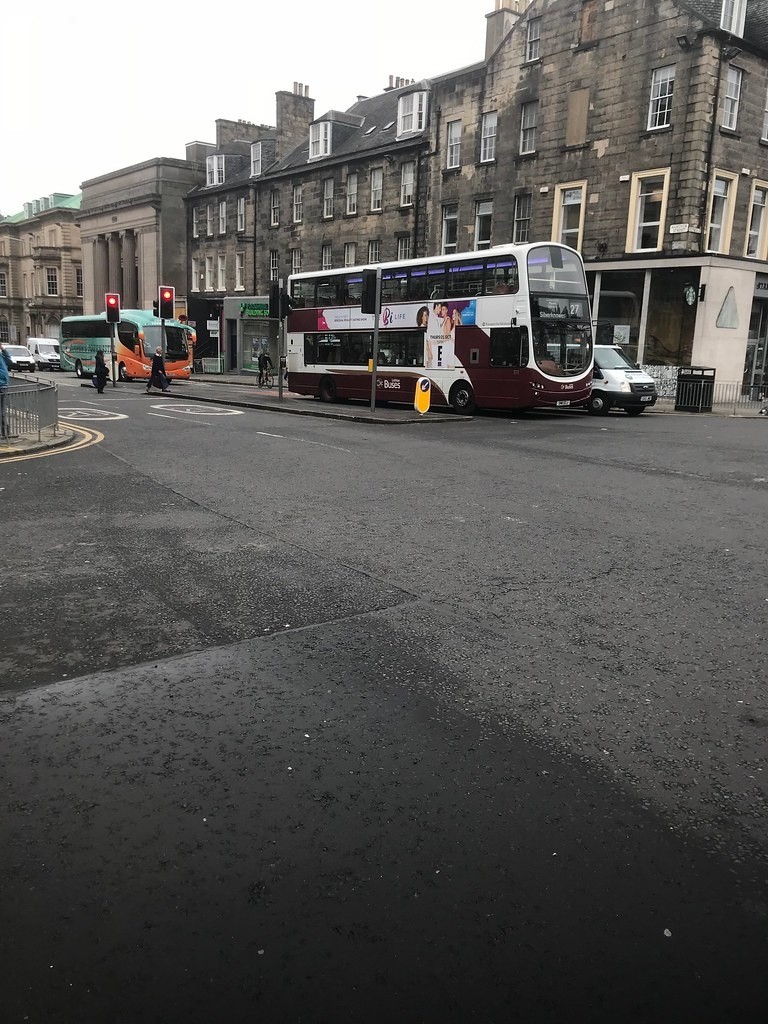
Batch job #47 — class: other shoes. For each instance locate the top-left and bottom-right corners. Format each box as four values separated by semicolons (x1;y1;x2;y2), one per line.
265;378;271;382
148;386;151;394
162;389;170;393
98;391;103;394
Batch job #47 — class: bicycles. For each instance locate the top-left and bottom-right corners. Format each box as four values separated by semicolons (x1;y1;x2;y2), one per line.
256;366;275;389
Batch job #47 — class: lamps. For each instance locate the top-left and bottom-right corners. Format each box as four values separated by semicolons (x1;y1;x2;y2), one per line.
722;47;743;61
676;34;693;51
384;155;396;166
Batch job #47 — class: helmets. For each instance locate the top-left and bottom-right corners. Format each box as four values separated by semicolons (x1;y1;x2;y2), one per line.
266;352;270;355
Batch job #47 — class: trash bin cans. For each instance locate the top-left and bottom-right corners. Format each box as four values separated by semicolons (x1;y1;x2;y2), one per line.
674;365;716;414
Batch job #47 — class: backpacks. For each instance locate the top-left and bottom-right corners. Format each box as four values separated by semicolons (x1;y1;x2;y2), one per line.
258;354;266;361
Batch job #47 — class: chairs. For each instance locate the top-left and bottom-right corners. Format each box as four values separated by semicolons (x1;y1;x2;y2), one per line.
291;281;495;308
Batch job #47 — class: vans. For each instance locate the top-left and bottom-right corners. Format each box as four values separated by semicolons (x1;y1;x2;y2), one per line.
27;337;60;372
0;344;35;374
548;342;658;417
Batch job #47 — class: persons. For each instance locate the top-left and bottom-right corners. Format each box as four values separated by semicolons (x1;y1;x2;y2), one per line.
416;302;462;368
147;346;171;392
574;331;591;343
0;335;21;440
258;352;275;385
95;351;109;393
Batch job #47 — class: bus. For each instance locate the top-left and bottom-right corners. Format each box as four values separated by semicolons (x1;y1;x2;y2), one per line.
586;289;639;344
59;310;198;383
286;242;594;417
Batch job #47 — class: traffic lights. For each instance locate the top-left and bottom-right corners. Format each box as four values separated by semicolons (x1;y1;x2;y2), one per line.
268;280;293;322
153;300;159;319
158;285;175;319
105;293;121;324
